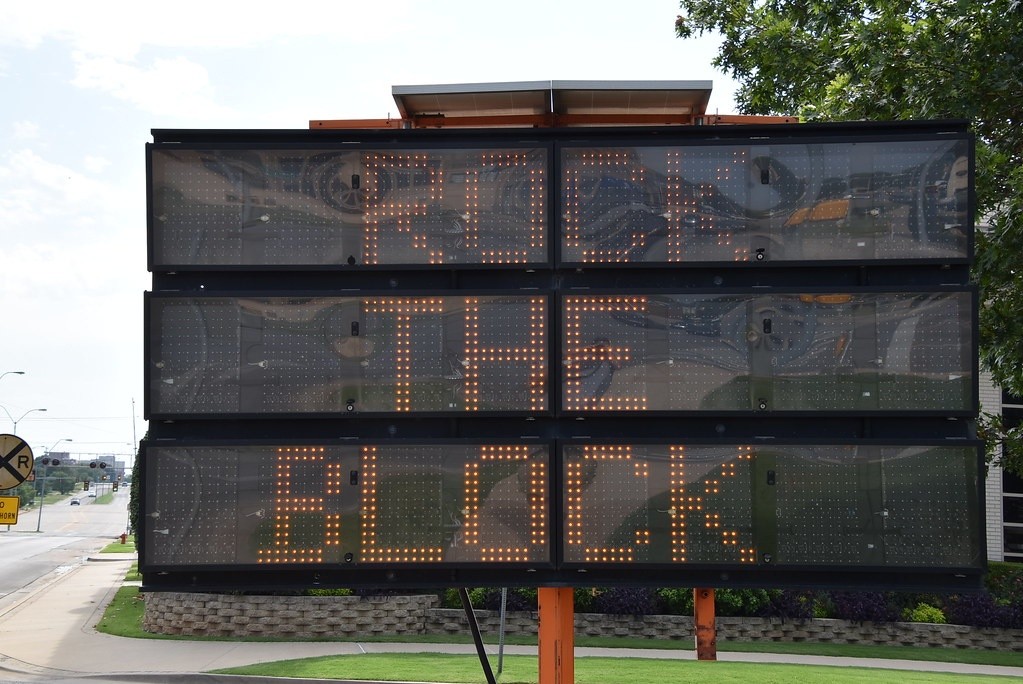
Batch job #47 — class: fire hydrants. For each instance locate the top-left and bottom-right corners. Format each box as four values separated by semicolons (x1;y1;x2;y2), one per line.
120;532;128;544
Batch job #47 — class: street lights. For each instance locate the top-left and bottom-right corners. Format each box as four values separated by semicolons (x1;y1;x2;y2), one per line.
0;404;47;436
34;438;73;533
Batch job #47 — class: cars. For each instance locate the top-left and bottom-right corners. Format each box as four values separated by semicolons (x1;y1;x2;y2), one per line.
122;481;128;487
88;491;96;497
70;497;80;505
